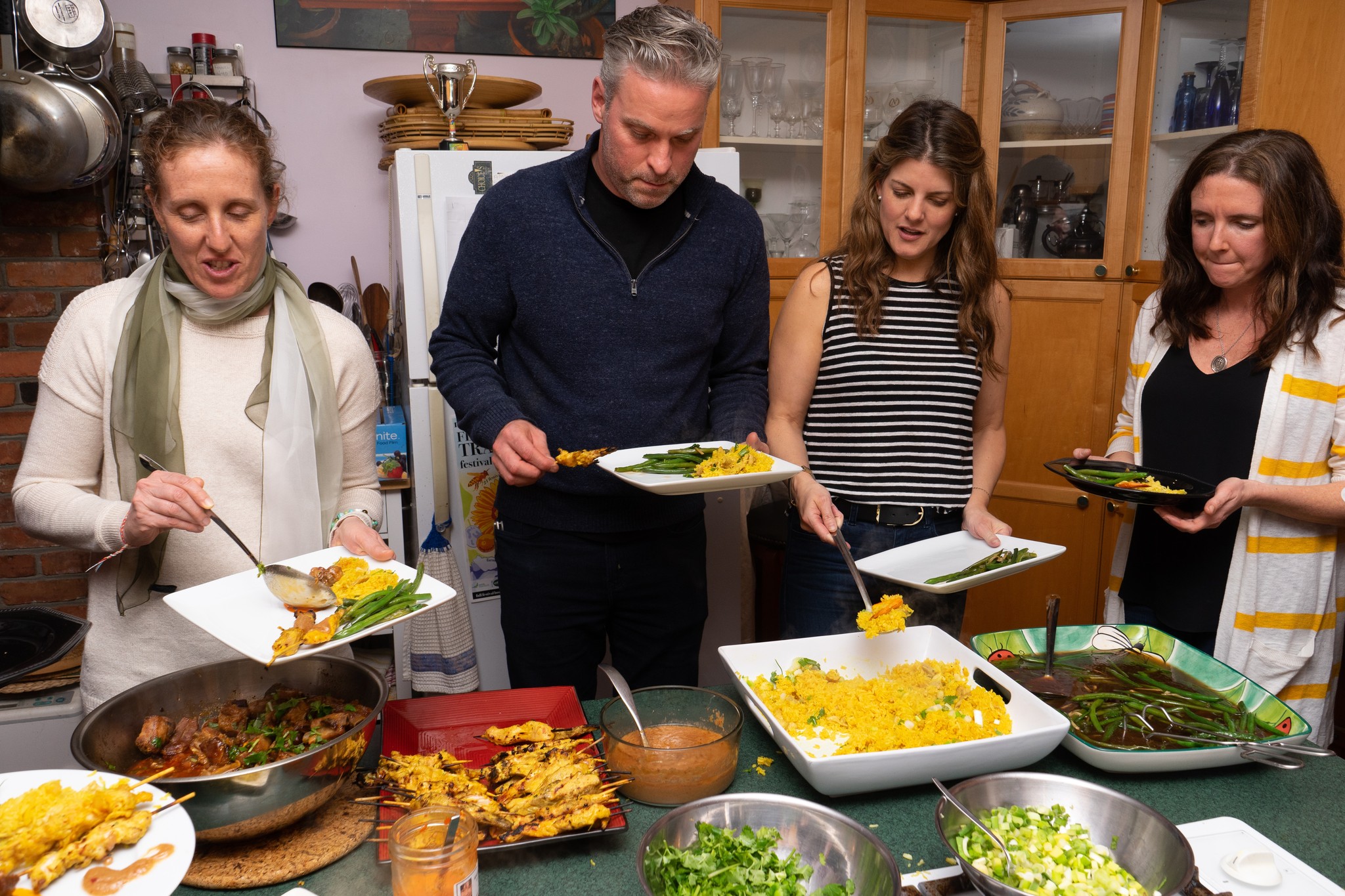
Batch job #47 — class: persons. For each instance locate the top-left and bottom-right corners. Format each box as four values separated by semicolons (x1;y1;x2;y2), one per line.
1071;128;1345;747
744;101;1012;640
429;5;769;703
12;98;397;716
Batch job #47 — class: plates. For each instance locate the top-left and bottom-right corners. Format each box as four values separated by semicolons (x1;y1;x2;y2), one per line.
162;546;457;666
592;440;804;496
1043;457;1217;507
854;530;1066;594
0;769;196;896
376;686;630;868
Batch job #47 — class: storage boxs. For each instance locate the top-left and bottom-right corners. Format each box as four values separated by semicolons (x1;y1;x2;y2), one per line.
375;406;408;481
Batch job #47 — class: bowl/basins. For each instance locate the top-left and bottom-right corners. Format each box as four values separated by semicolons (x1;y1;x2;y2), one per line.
1058;97;1103;140
970;624;1312;774
935;771;1194;896
718;625;1072;798
70;650;390;845
636;793;902;896
598;684;744;807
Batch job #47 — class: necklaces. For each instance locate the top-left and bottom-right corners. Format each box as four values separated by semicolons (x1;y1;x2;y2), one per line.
1210;298;1262;372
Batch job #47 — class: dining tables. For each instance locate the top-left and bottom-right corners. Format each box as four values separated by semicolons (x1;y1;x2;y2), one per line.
172;685;1345;896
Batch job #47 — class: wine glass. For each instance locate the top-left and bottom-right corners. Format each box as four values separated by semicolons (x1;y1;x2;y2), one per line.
1190;36;1247;130
741;178;820;258
720;54;936;142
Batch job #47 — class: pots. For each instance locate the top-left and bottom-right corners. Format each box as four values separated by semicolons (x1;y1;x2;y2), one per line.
0;0;124;193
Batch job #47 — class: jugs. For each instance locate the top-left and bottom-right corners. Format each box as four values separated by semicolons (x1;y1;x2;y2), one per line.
999;80;1064;142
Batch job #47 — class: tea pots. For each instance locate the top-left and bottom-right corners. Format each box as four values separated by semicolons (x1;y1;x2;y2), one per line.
1042;220;1104;260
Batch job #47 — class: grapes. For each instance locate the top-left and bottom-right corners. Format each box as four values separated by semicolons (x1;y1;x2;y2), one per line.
376;450;407;473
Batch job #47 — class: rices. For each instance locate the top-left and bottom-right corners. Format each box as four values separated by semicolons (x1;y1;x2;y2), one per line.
1129;475;1187;495
692;443;775;479
329;556;399;606
855;594;914;639
737;659;1013;758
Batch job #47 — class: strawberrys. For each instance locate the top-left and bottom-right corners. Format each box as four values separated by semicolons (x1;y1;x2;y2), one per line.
381;460;403;478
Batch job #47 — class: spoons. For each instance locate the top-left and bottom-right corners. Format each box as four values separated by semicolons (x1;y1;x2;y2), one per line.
931;776;1017;877
138;453;337;608
362;323;371;345
352;302;362;327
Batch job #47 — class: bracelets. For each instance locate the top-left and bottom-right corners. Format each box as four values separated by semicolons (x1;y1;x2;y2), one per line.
85;510;141;574
329;507;378;533
788;465;816;506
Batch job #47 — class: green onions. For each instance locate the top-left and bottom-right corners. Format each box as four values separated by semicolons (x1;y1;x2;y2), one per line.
957;803;1144;895
769;657;1001;744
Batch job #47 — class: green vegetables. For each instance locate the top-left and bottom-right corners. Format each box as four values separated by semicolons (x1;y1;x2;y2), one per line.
682;441;750;478
614;443;730;475
150;689;357;767
643;822;856;896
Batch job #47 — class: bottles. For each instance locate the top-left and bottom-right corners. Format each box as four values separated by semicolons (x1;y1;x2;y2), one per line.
1034;204;1062;258
1171;72;1197;132
1003;183;1037;259
191;33;217;75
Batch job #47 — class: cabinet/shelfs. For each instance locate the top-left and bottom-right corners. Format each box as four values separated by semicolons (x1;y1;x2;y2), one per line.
661;0;1345;649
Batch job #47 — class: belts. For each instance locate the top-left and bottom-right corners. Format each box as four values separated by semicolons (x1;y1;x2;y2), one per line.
834;498;961;526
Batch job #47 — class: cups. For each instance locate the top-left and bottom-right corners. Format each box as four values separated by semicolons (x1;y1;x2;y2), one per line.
1100;93;1116;138
371;350;402;424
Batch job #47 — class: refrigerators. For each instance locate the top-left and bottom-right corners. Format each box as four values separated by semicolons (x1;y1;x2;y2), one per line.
388;149;740;691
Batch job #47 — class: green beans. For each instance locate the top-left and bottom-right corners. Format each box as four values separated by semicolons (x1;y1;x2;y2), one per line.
922;548;1037;584
326;563;431;640
992;653;1288;749
1063;463;1149;485
